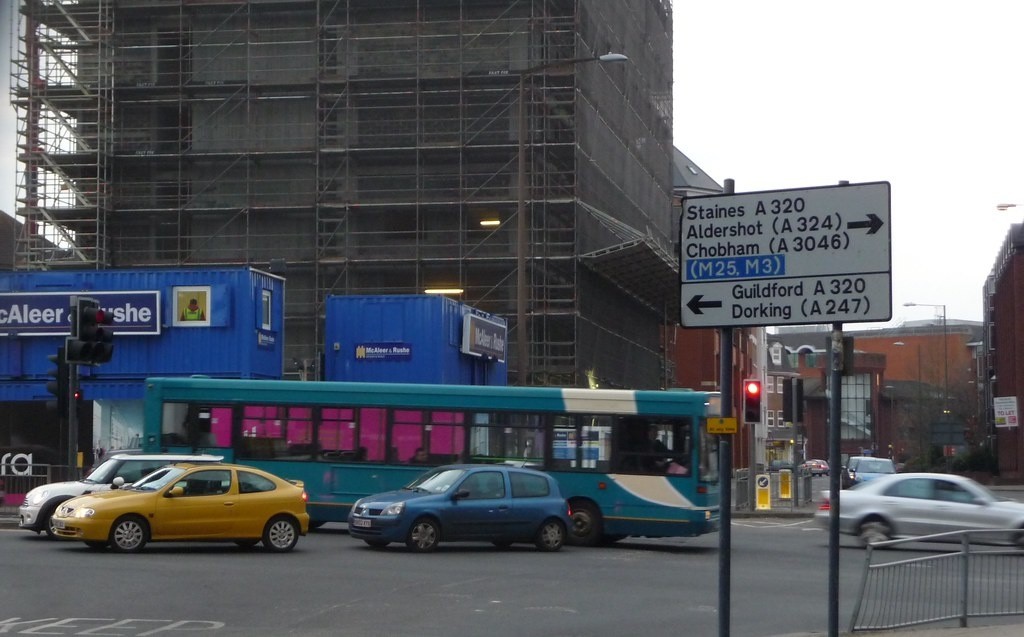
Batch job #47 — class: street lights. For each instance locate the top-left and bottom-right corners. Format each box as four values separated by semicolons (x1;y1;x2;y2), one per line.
904;302;949;465
895;341;923;470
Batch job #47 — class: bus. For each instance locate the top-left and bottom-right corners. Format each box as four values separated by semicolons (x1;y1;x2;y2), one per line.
142;374;726;548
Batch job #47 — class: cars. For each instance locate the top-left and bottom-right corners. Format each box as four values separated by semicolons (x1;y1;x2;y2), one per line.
348;465;572;553
813;472;1024;550
50;462;309;552
805;459;830;476
841;456;896;489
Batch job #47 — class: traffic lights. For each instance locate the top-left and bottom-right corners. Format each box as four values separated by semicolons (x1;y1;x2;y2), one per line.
65;296;114;365
743;378;762;424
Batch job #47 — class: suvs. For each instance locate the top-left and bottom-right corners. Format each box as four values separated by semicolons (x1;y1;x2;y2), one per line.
18;454;226;542
